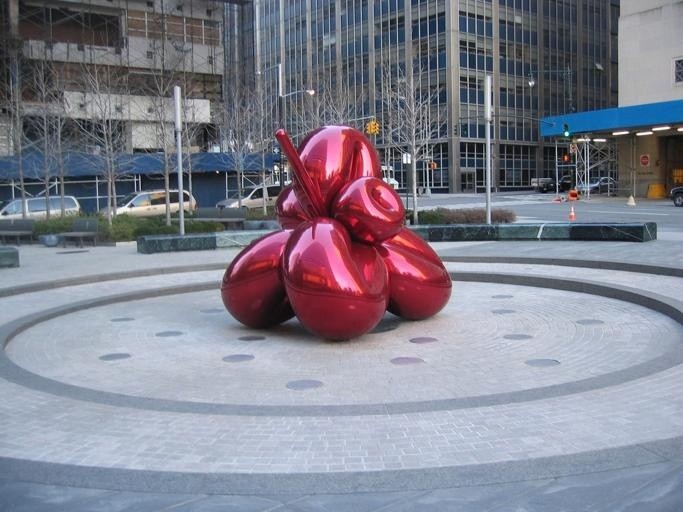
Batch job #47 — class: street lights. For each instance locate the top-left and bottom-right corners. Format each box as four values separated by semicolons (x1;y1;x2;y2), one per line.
277;89;315;188
528;69;575;115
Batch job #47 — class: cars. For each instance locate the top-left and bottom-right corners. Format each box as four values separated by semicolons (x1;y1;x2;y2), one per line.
670;186;683;207
544;175;572;194
577;177;617;194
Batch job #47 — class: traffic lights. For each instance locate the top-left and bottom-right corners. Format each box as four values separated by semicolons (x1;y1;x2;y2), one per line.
461;125;467;137
564;156;570;161
455;124;460;136
367;121;380;135
564;131;570;138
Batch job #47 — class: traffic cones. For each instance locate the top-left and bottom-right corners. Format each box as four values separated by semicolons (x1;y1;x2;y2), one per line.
569;206;576;222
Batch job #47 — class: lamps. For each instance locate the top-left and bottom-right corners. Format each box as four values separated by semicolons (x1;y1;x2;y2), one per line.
528;70;571;88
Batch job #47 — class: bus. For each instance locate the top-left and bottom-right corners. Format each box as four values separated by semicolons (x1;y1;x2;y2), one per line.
381;166;399;192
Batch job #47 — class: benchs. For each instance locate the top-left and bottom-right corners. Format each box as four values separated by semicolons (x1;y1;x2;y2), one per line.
56;219;103;248
0;219;36;247
191;208;247;230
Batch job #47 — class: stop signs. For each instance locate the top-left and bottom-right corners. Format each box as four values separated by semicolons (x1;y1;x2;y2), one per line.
640;156;649;166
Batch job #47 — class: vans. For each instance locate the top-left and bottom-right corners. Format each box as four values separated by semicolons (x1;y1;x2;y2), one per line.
215;184;281;210
102;189;197;221
0;196;82;220
